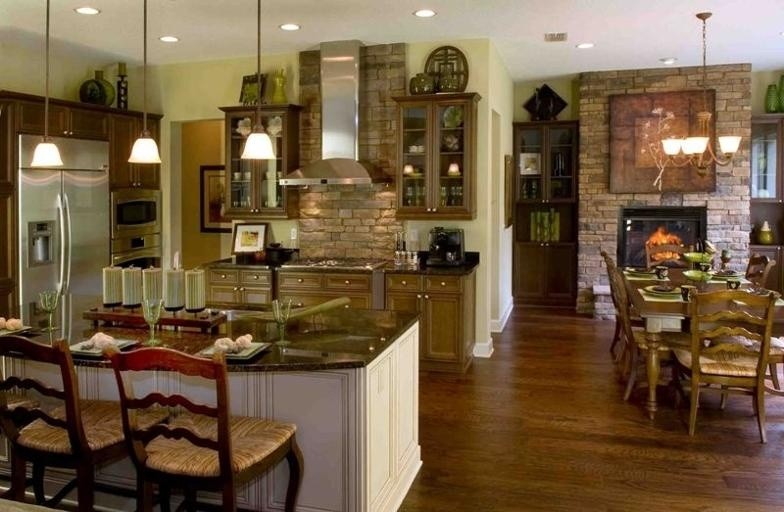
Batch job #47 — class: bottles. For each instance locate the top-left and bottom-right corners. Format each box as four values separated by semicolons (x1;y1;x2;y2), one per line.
396;264;418;272
396;250;419;265
441;185;463;207
759;220;774;244
762;75;784;112
552;150;568;176
405;186;425;206
521;180;538;200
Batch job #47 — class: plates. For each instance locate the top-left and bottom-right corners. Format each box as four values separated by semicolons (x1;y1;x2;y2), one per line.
0;322;33;338
442;105;465;127
68;335;141;357
628;268;657;275
711;269;745;278
644;285;684;295
193;339;274;361
445;172;463;177
741;287;783;299
408;171;424;176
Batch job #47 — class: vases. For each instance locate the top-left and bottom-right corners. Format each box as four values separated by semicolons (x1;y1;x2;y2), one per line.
764;84;780;113
778;75;784;112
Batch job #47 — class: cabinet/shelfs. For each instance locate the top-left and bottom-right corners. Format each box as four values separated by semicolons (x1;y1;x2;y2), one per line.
390;90;481;220
384;263;476;372
217;102;300;219
0;91;165;189
512;119;579;309
205;268;274;304
751;112;784;323
276;270;385;310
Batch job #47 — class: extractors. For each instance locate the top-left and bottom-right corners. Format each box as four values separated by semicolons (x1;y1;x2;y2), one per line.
277;42;393;187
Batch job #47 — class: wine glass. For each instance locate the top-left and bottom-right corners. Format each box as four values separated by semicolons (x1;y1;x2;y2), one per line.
38;291;58;332
139;298;163;347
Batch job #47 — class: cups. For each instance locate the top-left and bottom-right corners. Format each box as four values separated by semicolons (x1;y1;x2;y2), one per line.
680;286;698;301
232;172;250;181
701;263;713;271
656;266;669;279
727;278;742;289
265;171;282;179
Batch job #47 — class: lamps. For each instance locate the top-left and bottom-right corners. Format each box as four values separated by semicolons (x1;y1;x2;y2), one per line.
240;1;278;160
127;0;162;165
660;12;743;176
29;0;64;168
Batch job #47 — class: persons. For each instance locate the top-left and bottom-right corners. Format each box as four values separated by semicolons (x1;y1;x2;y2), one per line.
551;153;565;176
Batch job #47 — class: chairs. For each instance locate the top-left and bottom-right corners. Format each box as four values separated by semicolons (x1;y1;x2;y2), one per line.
102;343;303;511
0;335;172;511
1;395;43;500
600;248;784;444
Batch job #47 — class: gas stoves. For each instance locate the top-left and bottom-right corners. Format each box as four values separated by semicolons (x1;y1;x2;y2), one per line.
281;256;387;273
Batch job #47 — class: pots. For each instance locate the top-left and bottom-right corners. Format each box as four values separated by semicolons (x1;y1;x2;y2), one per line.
266;242;289;262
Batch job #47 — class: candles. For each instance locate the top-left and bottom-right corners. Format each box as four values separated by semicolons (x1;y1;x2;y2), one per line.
184;269;206;309
142;268;163;302
118;62;126;75
102;266;121;304
163;267;184;308
121;267;142;305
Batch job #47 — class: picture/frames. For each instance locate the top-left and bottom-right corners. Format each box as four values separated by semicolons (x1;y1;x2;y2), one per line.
505;155;514;228
520;152;542;175
200;165;232;233
230;222;268;254
238;73;268;102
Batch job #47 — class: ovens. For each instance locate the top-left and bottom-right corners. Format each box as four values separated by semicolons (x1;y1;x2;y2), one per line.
110;190;161;270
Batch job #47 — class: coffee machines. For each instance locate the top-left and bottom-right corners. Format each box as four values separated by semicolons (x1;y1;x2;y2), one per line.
426;226;467;266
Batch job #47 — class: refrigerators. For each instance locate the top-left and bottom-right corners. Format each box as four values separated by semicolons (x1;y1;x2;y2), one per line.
18;136;114;329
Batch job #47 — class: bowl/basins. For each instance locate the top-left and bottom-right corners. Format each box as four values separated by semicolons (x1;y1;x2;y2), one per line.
683;251;715;262
407;145;425;153
232;200;252;207
682;269;713;281
